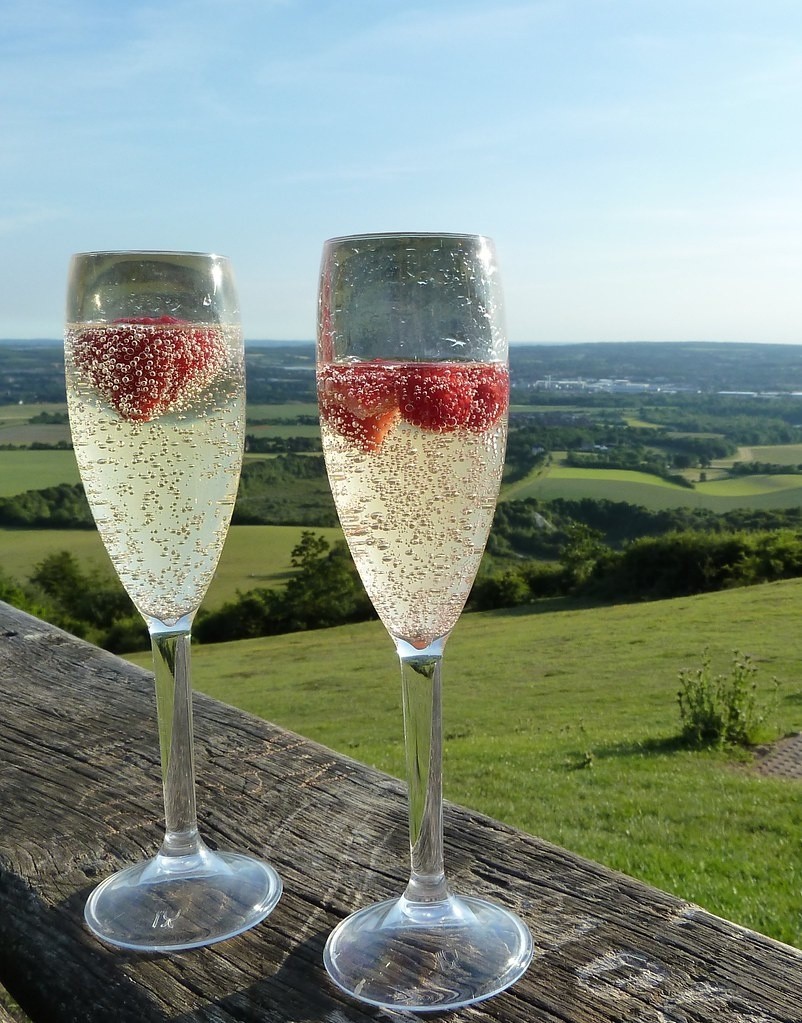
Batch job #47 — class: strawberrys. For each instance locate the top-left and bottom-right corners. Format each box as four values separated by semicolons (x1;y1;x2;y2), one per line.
317;363;509;457
77;316;221;424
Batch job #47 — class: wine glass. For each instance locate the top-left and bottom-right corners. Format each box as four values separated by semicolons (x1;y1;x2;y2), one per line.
316;232;532;1010
62;250;282;952
